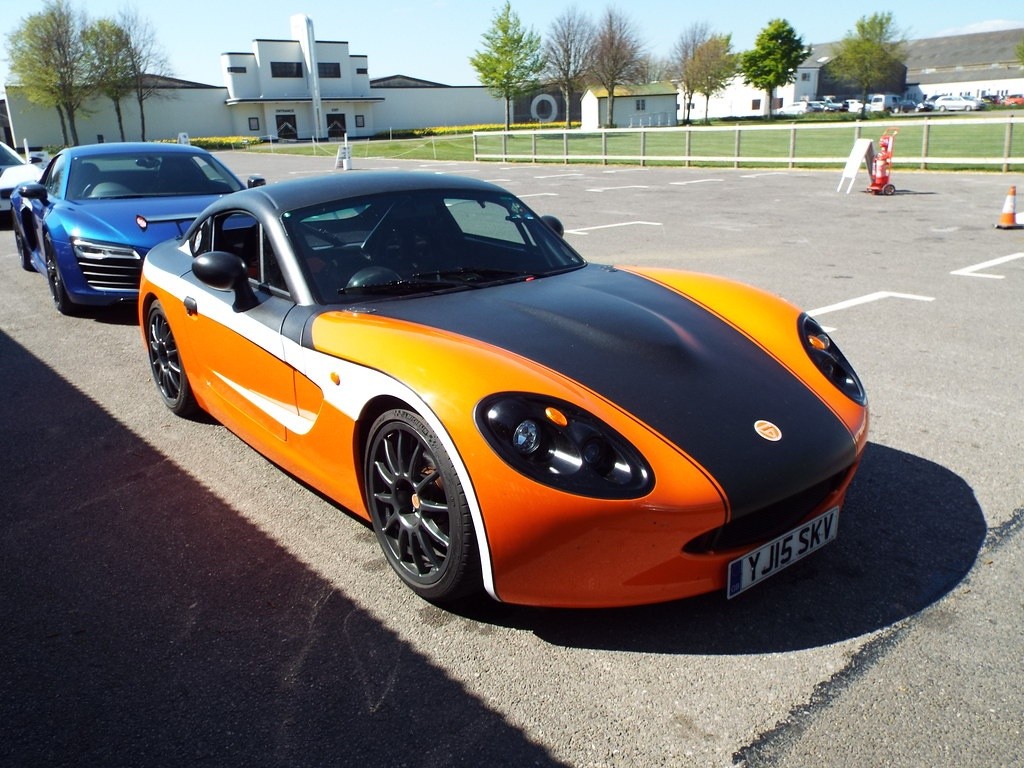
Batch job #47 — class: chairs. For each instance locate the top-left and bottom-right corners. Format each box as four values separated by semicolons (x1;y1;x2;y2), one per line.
313;243;378;304
429;232;481;286
74;162;103;195
160;158;204;191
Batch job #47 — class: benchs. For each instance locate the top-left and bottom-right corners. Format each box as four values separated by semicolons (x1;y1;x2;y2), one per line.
99;169;157;192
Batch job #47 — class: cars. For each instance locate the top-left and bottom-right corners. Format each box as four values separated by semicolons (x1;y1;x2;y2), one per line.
917;95;945;112
900;99;917;113
1000;98;1005;104
811;103;834;113
981;97;991;104
963;97;986;108
773;102;810;115
1004;94;1024;106
846;100;871;112
868;94;872;100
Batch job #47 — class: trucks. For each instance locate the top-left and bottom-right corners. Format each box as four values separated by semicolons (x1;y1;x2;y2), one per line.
809;96;849;113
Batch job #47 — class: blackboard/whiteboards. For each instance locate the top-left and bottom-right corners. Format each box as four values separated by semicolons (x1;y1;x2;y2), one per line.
335;145;351;167
842;139;872;179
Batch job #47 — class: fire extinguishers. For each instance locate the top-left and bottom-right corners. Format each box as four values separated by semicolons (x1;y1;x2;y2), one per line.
871;151;887;185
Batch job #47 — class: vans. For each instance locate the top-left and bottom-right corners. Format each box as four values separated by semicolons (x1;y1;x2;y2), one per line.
871;95;902;113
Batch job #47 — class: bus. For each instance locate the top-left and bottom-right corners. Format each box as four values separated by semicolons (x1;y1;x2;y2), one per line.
982;95;999;104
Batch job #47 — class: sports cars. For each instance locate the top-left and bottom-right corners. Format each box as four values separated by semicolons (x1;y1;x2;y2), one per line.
136;176;872;624
9;143;266;314
0;141;45;227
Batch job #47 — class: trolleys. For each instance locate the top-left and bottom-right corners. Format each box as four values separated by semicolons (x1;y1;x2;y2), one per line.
866;126;900;194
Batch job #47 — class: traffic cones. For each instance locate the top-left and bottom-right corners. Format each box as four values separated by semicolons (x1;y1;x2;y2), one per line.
993;185;1024;229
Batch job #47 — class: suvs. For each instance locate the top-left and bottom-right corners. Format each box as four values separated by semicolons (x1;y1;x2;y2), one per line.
934;96;979;111
